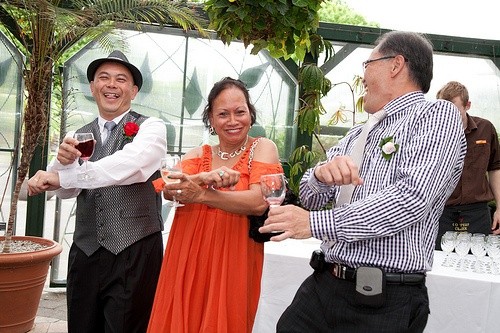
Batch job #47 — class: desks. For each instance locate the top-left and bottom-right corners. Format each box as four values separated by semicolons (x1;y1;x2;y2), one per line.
251;236;500;333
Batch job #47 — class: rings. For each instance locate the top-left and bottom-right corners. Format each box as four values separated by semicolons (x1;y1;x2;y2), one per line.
177;189;182;196
34;178;39;183
220;171;225;176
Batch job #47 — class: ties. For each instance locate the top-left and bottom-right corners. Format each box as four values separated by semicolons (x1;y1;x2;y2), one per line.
329;109;388;247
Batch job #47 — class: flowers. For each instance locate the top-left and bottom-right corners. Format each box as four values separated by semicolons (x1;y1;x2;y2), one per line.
122;120;138;142
379;133;400;159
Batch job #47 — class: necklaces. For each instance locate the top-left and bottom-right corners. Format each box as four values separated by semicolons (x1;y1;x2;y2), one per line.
217;134;248;160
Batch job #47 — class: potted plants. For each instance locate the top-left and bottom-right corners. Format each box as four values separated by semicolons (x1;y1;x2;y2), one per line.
0;0;208;333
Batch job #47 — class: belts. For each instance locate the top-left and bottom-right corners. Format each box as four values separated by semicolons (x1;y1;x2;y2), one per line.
322;262;425;284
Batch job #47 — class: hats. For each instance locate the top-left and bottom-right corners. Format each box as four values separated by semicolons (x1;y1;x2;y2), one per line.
87;50;143;92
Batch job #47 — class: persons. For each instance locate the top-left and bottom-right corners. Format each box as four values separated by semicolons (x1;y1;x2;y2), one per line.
259;31;467;333
435;81;499;257
146;76;284;333
27;50;167;333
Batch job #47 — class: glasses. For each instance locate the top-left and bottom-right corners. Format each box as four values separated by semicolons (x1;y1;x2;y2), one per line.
362;55;408;72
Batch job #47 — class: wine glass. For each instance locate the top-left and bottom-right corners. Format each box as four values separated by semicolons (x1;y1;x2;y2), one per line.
441;231;500;276
160;156;185;207
74;132;96;181
260;173;284;233
